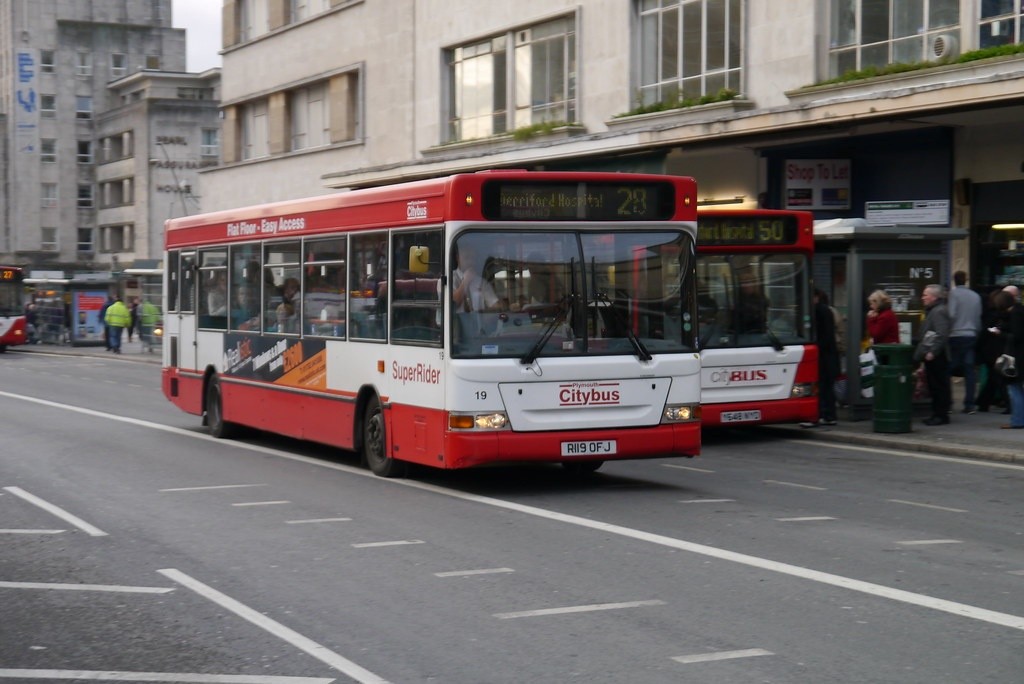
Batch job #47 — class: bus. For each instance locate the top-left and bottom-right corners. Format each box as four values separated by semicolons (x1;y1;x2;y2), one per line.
587;207;819;426
160;165;703;486
0;266;29;352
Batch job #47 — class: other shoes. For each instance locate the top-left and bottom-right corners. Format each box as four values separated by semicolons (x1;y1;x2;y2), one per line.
923;413;949;425
800;422;819;427
818;417;836;425
962;406;977;415
1002;423;1022;429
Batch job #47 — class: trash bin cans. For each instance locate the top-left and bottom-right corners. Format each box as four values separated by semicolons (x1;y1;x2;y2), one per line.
870;343;917;434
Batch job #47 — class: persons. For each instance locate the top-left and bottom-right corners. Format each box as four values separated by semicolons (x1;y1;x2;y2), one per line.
170;253;344;333
26;296;71;345
500;253;566;311
735;273;767;332
574;250;630;335
352;270;384;296
436;245;502;327
800;287;841;428
697;284;719;324
866;270;1024;429
98;295;161;353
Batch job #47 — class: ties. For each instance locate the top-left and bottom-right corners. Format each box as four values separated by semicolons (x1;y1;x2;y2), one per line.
462;277;472;313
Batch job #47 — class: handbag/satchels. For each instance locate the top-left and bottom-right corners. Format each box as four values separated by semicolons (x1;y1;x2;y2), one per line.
996;354;1019;377
913;361;931;400
